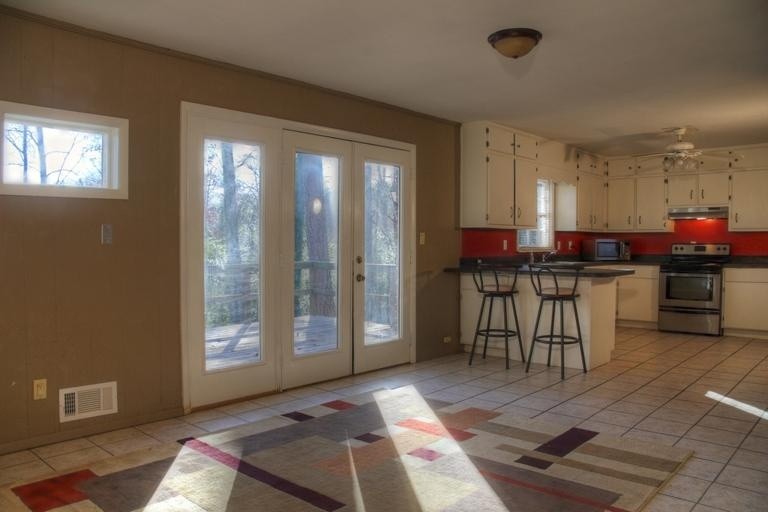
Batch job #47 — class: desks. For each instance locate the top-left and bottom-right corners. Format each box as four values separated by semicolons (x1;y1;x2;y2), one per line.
442;262;635;373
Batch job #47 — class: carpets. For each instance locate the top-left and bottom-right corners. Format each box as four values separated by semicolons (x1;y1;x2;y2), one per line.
2;379;696;512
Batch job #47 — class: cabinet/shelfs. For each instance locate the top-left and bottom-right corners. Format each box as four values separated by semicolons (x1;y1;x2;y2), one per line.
727;145;767;232
595;264;659;329
553;173;608;233
576;150;608;177
718;265;768;340
457;147;539;231
665;148;727;207
458;119;540;160
607;173;673;234
607;152;663;179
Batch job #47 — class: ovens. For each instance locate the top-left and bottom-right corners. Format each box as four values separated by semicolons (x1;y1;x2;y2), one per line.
660;271;721;335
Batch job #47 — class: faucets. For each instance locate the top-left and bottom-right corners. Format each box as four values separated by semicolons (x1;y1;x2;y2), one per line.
542;250;556;263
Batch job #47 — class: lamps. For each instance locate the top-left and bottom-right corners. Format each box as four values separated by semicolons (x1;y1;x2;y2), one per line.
661;151;700;174
485;27;543;61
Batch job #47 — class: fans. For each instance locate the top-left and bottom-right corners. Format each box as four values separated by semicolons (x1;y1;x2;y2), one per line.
623;124;745;171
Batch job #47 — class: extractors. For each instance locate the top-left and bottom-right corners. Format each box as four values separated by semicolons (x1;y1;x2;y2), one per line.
667;207;729;219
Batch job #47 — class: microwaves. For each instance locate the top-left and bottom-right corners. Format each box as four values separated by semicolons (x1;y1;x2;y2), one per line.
581;238;631;262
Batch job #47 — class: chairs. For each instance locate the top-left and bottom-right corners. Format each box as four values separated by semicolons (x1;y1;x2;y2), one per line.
523;257;589;380
467;259;526;370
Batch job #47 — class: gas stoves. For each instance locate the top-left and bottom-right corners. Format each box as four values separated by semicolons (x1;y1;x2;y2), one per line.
662;255;728;270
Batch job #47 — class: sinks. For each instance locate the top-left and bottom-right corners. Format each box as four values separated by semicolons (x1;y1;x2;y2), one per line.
528;263;573;270
